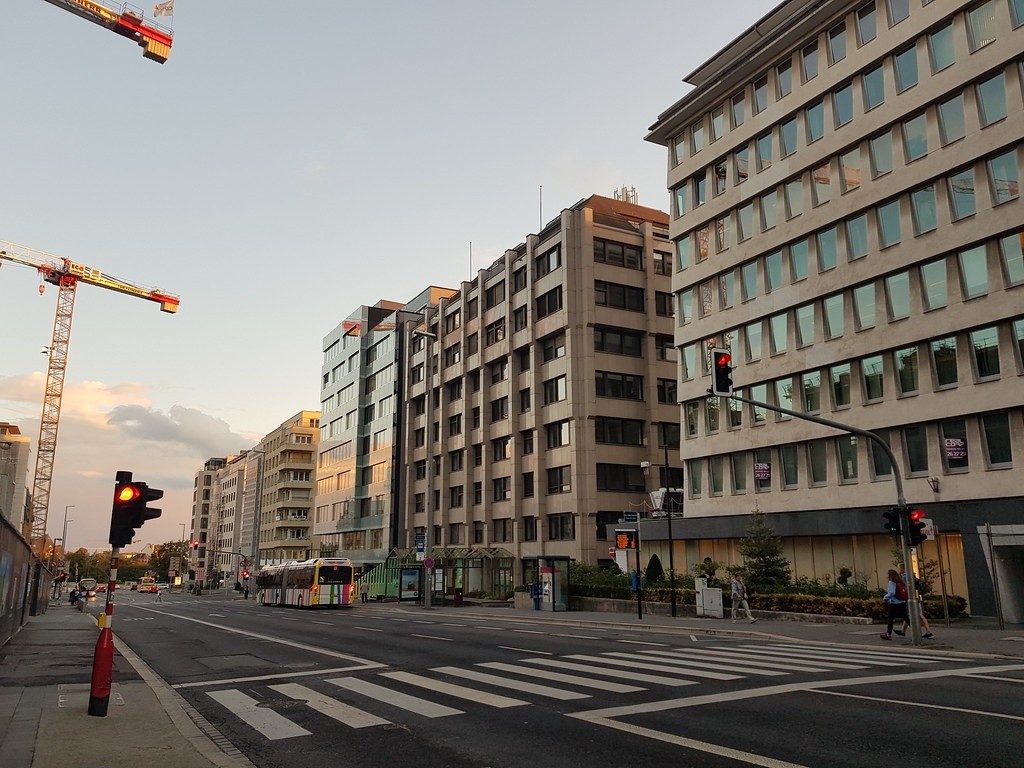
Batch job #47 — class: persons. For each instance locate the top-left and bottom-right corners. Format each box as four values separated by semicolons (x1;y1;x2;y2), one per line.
155;589;163;603
880;569;912;641
360;580;369;603
893;562;935;640
244;585;249;600
85;590;91;601
729;573;759;623
70;589;82;606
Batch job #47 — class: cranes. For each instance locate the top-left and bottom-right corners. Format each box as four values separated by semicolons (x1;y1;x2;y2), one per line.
43;1;178;65
0;238;182;563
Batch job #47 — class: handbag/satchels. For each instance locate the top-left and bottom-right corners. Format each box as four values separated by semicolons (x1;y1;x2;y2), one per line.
918;583;930;596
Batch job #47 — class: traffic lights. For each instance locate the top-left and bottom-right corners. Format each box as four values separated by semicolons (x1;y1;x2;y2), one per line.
904;506;928;547
193;540;199;551
109;482;144;548
883;509;900;534
134;482;164;529
242;570;250;580
710;348;733;397
59;572;67;583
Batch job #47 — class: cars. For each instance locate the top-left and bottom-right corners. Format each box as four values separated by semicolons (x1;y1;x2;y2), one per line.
129;570;169;593
115;583;120;589
96;585;106;593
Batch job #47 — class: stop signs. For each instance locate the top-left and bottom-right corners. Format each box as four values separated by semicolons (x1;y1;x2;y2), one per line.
609;549;615;558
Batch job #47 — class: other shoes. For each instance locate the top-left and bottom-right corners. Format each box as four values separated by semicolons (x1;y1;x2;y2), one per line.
922;632;934;639
751;618;758;625
893;628;906;637
879;632;892;641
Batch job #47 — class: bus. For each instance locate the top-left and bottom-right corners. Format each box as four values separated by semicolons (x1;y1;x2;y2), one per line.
78;577;97;597
256;556;355;609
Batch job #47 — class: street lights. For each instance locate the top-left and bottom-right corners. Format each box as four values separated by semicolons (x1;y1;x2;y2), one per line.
61;505;76;556
411;328;437;609
63;519;74;549
252;450;266;599
639;445;677;617
179;523;186;577
50;538;62;573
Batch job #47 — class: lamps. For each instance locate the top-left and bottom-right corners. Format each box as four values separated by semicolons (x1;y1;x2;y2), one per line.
927;477;939;494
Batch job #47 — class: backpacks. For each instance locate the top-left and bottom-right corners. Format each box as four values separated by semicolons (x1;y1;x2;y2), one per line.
892;580;908;601
